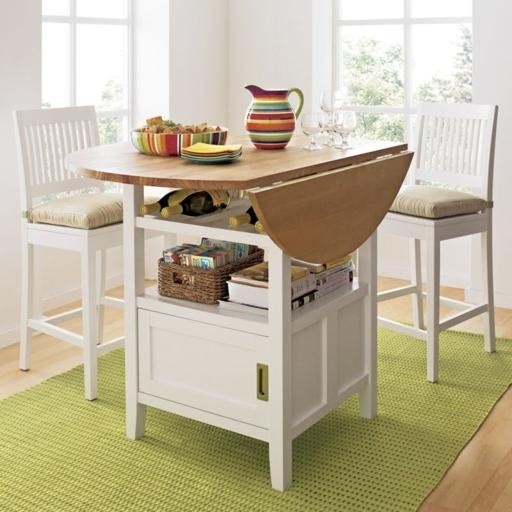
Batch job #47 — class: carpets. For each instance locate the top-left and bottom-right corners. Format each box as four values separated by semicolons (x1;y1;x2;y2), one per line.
0;322;511;511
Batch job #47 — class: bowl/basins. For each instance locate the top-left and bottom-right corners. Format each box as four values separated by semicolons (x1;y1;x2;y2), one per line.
129;128;228;156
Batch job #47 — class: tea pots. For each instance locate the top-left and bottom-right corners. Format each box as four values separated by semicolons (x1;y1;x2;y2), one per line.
244;84;304;149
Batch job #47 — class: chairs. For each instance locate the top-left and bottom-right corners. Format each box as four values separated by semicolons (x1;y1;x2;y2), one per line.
377;102;497;384
16;107;176;401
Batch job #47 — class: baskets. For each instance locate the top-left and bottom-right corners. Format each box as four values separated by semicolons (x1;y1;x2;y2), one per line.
155;237;267;307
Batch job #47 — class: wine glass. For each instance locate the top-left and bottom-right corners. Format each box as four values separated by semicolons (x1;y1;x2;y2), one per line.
299;90;356;152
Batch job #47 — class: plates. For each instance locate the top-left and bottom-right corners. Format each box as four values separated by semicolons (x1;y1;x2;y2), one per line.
179;142;244;162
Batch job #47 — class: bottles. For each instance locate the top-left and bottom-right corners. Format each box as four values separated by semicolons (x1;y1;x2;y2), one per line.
139;188;262;231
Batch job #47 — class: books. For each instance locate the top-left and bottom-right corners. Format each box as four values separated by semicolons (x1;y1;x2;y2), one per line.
225;274;315;308
217;290;318;316
162;237;259;287
230;261;309;287
291;257;356;297
